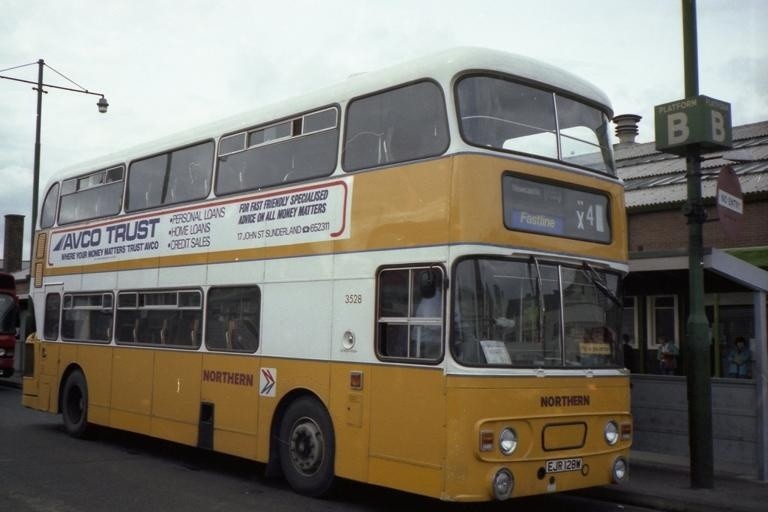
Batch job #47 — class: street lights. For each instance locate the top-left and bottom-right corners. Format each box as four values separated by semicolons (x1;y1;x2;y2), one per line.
0;59;109;376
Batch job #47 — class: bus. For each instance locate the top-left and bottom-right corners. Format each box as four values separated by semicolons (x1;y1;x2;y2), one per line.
21;44;634;504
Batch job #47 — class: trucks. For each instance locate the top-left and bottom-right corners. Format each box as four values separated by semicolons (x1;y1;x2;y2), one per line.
0;271;20;378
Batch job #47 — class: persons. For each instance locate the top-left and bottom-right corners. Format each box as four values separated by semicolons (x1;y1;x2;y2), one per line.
655;334;679;375
727;335;750;379
